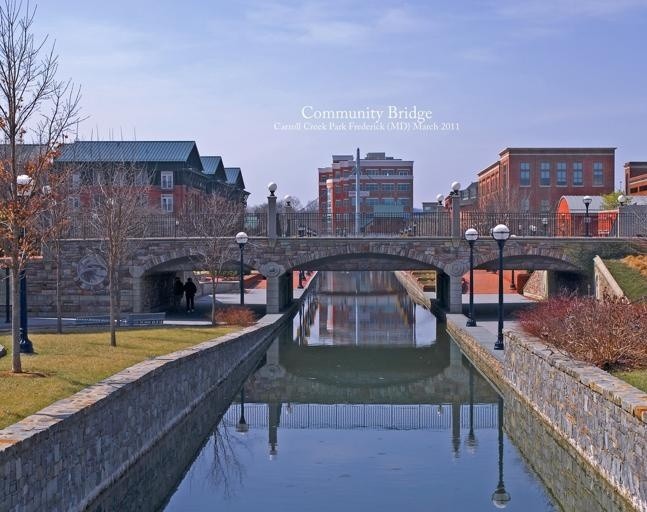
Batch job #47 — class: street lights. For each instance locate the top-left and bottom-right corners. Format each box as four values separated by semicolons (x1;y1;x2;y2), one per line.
16;176;34;353
235;233;249;308
582;196;592;236
518;218;548;235
493;223;510;351
439;387;511;510
618;195;625;205
296;224;317;289
234;391;293;460
465;229;478;327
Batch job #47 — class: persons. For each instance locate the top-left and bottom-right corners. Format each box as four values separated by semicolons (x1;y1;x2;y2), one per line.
173;277;184;307
183;276;198;314
